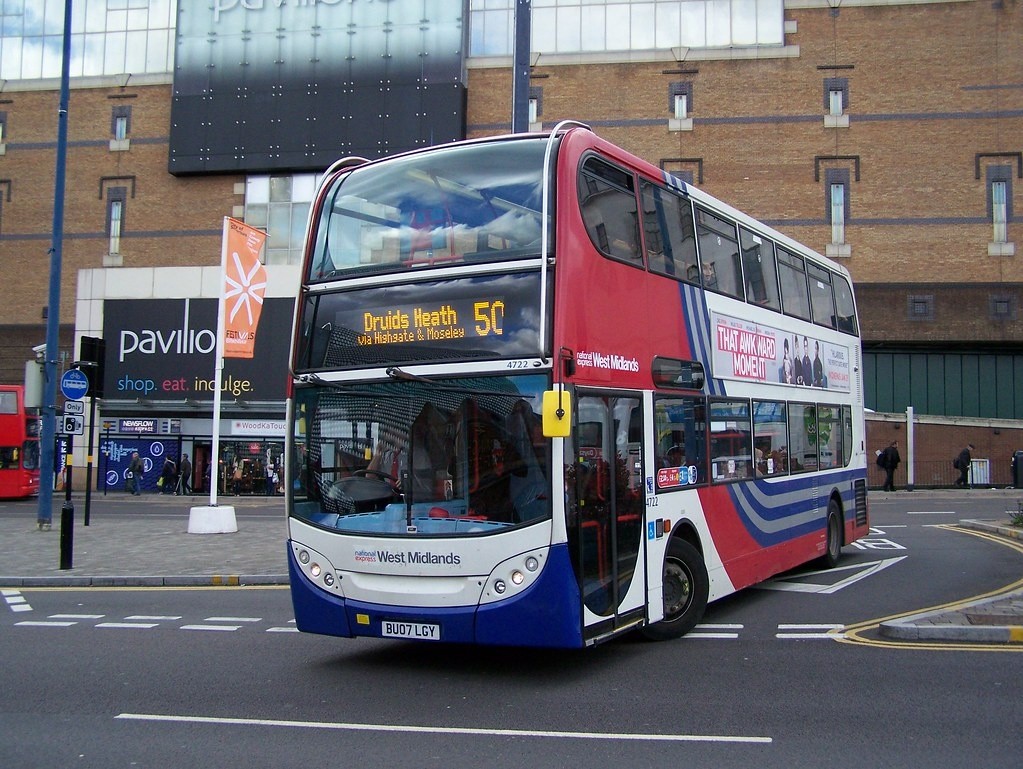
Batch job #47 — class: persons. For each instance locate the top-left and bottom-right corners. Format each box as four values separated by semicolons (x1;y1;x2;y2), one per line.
835;297;853;333
802;336;812;386
701;259;712;287
366;424;436;501
954;442;977;488
180;453;194;495
233;453;244;497
813;341;823;386
882;440;901;492
205;458;224;495
266;457;278;496
793;335;803;385
783;339;793;384
127;452;144;495
159;454;177;496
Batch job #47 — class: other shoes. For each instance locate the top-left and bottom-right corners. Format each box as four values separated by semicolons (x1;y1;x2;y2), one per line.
955;482;960;488
891;489;896;491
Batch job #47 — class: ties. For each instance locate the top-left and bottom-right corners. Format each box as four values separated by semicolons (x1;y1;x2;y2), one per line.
390;447;402;496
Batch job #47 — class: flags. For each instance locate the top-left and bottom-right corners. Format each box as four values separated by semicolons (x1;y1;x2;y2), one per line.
222;217;266;358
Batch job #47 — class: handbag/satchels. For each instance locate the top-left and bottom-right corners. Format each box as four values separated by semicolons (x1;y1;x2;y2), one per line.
127;472;133;478
876;454;886;468
953;458;959;468
272;473;279;483
157;477;164;486
233;469;243;480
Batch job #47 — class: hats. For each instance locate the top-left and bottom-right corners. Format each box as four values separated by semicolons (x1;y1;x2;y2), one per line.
969;444;975;449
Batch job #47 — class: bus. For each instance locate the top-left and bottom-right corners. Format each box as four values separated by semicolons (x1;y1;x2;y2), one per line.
0;384;41;499
282;119;871;658
